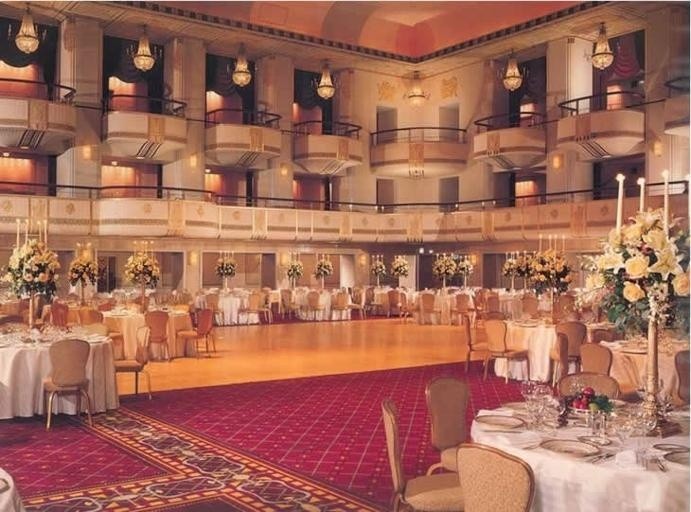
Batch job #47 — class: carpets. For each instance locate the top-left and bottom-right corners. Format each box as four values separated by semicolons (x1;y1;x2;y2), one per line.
0;356;553;512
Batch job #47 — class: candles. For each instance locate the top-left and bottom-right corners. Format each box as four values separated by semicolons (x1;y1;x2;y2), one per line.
612;173;626;238
37;220;42;243
16;218;21;250
635;175;644;212
44;218;47;249
26;219;30;245
660;171;671;240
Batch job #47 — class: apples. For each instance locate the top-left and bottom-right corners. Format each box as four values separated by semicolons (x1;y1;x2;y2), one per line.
567;387;599;410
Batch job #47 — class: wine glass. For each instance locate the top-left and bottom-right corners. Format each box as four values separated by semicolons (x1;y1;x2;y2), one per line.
613;405;656;456
519;381;566;436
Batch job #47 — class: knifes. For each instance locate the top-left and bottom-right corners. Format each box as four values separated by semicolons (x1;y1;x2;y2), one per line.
483;430;522;434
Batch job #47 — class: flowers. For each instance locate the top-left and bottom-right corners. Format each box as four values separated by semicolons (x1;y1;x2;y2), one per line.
571;208;691;336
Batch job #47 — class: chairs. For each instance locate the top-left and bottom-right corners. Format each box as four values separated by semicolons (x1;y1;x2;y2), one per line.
0;284;221;432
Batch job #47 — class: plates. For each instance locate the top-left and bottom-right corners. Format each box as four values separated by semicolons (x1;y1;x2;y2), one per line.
569;399;627;415
577;434;611;447
539;439;601;459
474;414;525;429
653;443;688;453
662;451;689;468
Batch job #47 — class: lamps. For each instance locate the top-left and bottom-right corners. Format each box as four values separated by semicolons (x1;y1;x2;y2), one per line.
229;42;253;86
132;22;157;72
586;21;616;73
408;70;426;107
315;58;336;100
502;48;524;91
12;1;42;56
